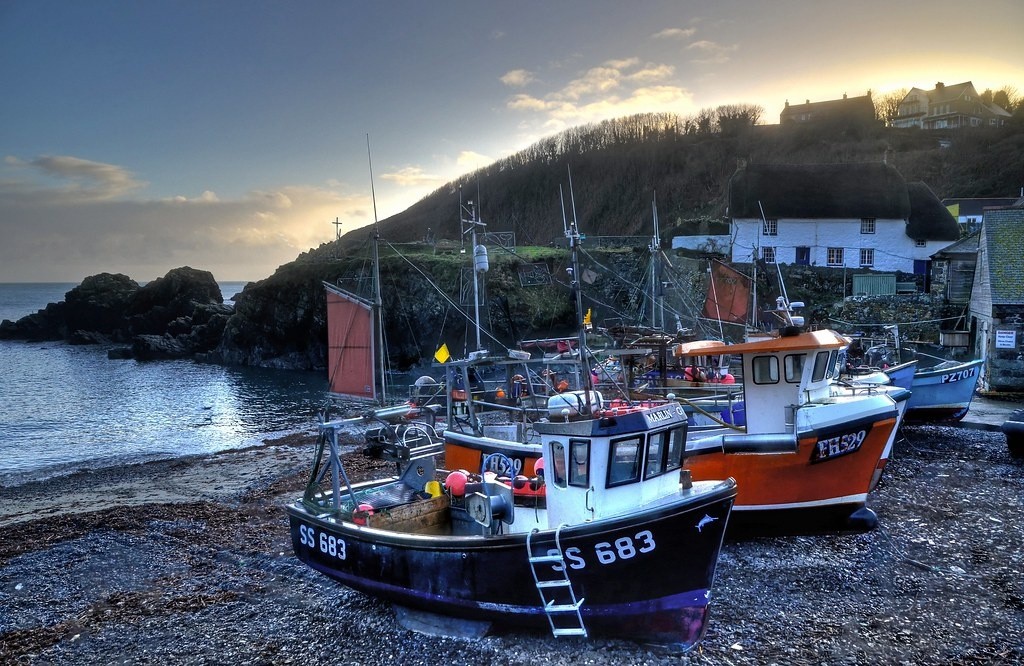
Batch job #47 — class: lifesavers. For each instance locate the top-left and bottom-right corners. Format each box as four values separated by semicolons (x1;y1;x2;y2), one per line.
541;369;557;391
596;406;646;416
509;374;527;398
413;376;436;405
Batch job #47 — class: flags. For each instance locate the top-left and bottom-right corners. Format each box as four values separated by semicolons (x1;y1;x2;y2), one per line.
435;344;450;364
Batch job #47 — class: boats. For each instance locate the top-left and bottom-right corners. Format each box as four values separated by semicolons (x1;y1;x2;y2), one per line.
404;160;985;540
286;129;740;655
